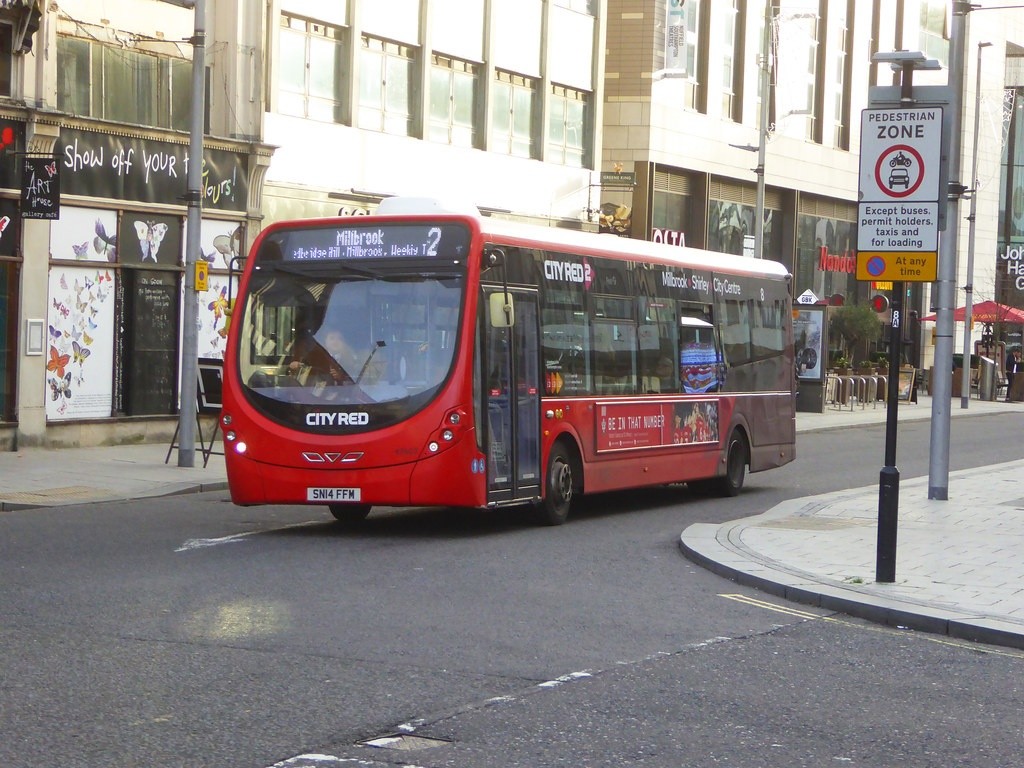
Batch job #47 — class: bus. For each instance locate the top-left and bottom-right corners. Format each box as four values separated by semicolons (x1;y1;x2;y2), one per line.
221;198;798;525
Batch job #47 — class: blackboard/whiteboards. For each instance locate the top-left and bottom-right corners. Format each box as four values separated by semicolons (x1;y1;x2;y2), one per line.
197;364;223;408
19;158;62;220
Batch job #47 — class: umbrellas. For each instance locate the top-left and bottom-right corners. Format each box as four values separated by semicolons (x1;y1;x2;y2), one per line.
915;301;1024;360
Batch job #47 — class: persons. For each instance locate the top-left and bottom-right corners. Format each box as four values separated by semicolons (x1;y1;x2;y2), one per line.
651;353;675;393
287;326;357;382
1005;348;1022;401
